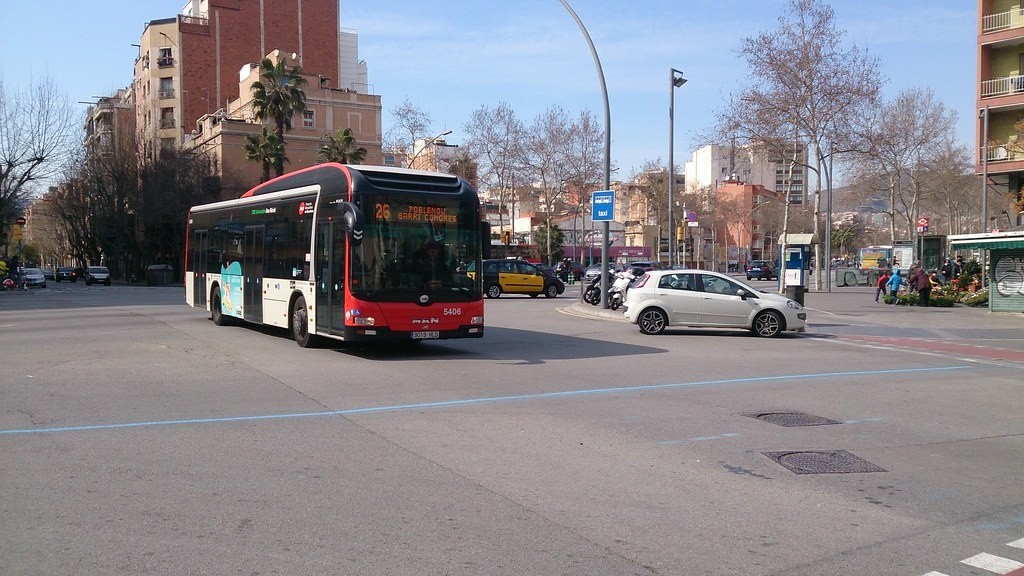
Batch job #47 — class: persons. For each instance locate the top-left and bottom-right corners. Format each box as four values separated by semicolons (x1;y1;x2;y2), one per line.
908;268;931;306
941;258;953;282
418;246;446;278
728;263;738;271
929;272;938;288
0;255;21;287
875;272;891;302
952;255;965;279
907;260;920;293
744;263;747;273
885;269;908;305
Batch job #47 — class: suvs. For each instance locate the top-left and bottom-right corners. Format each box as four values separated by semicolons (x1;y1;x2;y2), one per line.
746;259;778;281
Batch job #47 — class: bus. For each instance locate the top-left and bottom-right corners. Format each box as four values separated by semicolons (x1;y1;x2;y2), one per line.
489;240;544;263
184;162;492;349
858;246;892;268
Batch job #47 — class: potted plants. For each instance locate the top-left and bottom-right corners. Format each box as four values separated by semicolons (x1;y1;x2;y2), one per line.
883;294;956;307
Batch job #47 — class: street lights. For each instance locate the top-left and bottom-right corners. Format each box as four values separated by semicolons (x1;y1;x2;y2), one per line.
978;105;990;288
826;141;839;293
668;67;688;269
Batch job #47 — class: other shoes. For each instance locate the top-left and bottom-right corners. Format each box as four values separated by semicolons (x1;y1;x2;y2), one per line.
896;299;900;304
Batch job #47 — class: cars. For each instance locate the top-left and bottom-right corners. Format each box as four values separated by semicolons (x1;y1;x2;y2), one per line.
18;267;46;289
585;262;618;282
549;261;585;282
86;265;112;286
621;269;807;339
809;256;857;271
462;258;566;300
582;260;718;312
42;269;54;281
56;267;78;282
526;263;556;276
